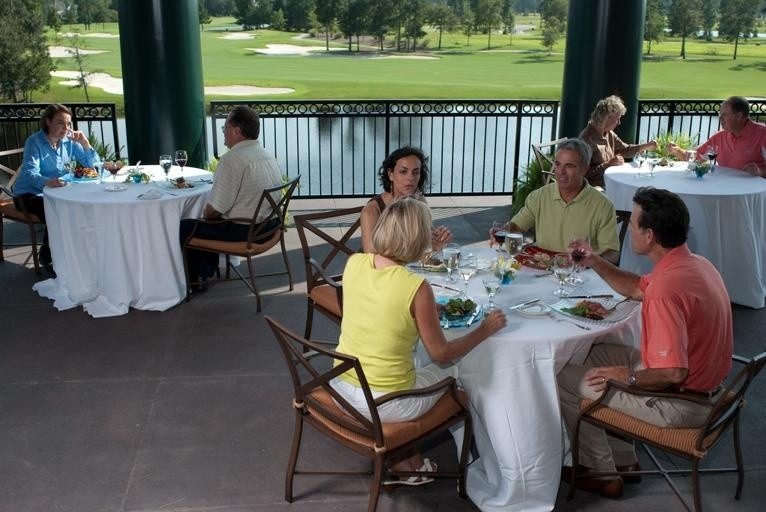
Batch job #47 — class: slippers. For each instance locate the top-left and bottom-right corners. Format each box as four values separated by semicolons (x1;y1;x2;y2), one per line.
414;458;437;473
382;469;435;486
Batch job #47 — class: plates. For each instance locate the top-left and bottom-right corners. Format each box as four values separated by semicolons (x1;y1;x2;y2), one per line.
437;297;483;330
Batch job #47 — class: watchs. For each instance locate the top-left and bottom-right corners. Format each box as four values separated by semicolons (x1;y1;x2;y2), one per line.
627;371;637;386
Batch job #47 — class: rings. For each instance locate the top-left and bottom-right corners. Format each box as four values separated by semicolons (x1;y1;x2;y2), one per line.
602;377;606;382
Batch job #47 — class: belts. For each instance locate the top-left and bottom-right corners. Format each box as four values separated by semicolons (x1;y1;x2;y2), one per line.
673;386;721;398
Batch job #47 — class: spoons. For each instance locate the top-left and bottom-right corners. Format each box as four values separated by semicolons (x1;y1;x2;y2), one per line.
544;310;592;331
441;311;449;329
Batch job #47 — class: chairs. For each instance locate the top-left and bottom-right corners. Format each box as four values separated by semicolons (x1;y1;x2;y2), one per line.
262;314;473;512
532;137;570;185
0;147;46;277
569;351;765;512
182;174;301;313
614;210;632;266
293;205;365;362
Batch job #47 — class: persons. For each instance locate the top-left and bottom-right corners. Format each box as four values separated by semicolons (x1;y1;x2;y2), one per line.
665;95;766;176
579;95;659;186
361;147;454;255
13;104;100;279
490;138;621;268
180;105;282;290
330;197;508;485
555;187;734;500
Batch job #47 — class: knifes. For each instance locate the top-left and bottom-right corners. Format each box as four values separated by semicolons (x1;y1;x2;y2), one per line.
558;294;613;299
466;305;483;327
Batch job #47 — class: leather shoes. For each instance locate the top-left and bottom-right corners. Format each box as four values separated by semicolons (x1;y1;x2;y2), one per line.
615;462;642;485
561;469;623;500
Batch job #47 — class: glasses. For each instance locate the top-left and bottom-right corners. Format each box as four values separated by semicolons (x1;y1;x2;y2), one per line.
221;126;235;131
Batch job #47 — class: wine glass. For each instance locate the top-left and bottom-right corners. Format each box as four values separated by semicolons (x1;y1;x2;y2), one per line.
631;126;718;179
415;221;590;310
63;150;188;192
